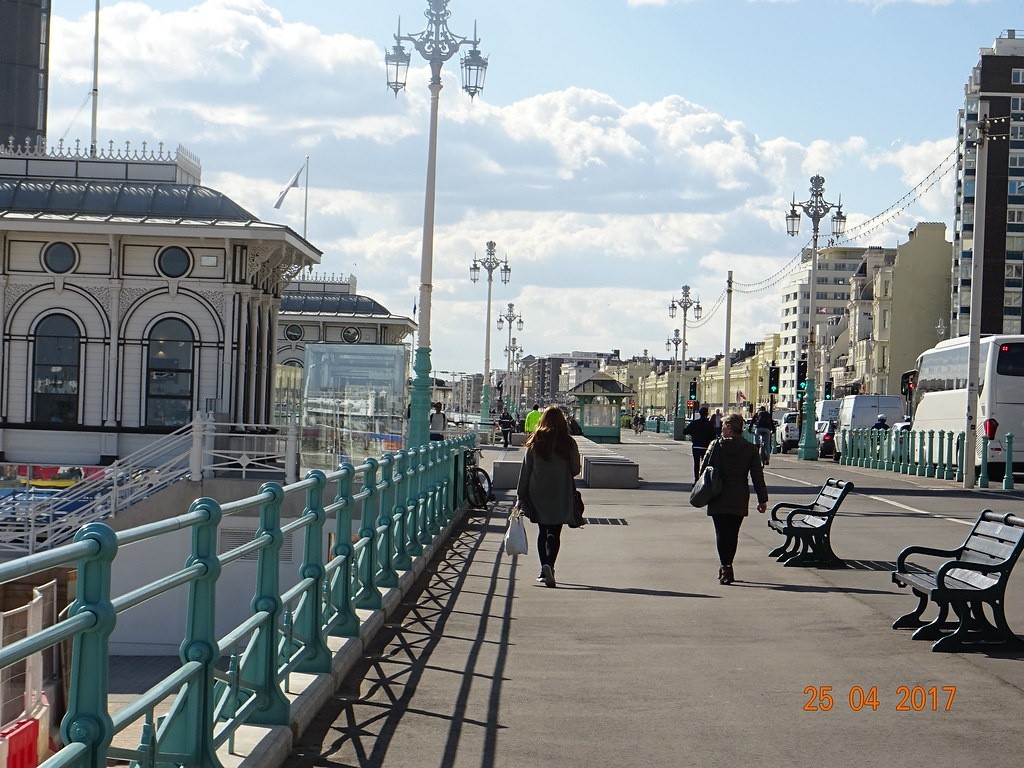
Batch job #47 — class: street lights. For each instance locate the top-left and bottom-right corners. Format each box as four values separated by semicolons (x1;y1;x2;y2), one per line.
497;302;524;413
665;327;689;419
468;239;512;427
382;0;488;450
503;337;526;415
785;172;847;462
669;285;703;416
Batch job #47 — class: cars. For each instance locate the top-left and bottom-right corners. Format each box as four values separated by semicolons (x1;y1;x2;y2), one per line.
742;411;838;459
881;421;911;463
646;414;664;421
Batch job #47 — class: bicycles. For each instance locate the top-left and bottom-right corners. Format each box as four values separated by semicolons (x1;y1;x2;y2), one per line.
632;422;642;435
458;445;497;510
748;431;774;469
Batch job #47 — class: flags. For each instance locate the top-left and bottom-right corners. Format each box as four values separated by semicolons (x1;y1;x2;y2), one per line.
274;158;308;209
413;296;416;314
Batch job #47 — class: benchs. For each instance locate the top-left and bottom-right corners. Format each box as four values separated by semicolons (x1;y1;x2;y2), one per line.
892;509;1024;653
768;478;854;567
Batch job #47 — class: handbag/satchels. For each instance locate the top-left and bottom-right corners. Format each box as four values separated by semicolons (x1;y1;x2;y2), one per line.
567;490;586;528
503;508;528;556
689;466;722;508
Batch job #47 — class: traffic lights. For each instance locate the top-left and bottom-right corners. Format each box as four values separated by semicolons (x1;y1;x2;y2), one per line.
797;392;802;398
689;381;696;401
797;360;807;391
825;382;832;400
768;366;780;395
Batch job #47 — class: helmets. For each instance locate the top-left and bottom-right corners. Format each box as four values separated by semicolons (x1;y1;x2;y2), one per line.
878;414;886;419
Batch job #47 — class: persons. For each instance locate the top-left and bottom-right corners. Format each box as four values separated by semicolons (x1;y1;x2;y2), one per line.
870;414;890;445
631;414;645;432
489;404;543;447
564;416;582;435
430;402;447;441
515;405;580;588
699;414;768;585
710;409;723;438
749;406;776;465
683;407;716;487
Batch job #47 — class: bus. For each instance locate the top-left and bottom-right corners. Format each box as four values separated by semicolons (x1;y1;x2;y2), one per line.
900;333;1024;475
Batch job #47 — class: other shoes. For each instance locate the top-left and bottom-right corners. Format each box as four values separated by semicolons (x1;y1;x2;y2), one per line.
542;564;556;587
537;573;546;582
765;460;769;465
718;564;735;585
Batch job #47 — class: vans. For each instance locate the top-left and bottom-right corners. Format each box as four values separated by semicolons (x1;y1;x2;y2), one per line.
815;395;904;462
